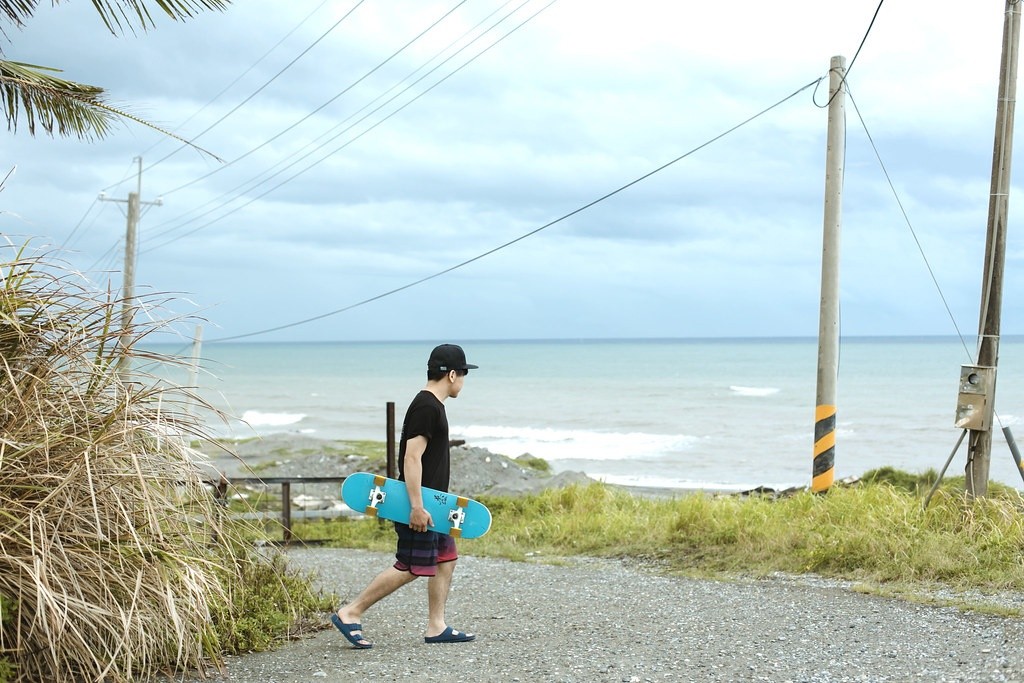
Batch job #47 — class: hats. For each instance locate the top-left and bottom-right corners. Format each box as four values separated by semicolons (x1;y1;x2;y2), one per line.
428;344;478;371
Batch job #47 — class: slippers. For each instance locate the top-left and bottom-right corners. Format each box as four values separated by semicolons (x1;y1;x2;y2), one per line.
425;626;475;643
331;613;373;648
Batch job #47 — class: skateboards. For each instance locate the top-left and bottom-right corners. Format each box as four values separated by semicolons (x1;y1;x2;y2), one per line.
338;472;492;541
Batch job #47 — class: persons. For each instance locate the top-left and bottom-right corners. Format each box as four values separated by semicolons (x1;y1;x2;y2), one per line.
332;343;478;648
